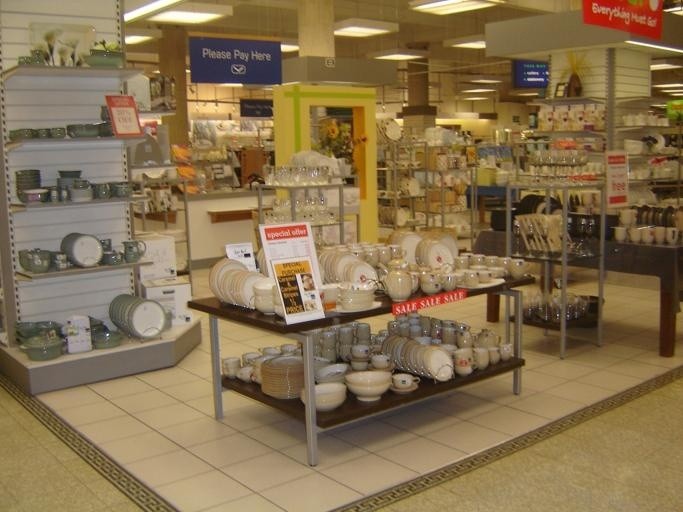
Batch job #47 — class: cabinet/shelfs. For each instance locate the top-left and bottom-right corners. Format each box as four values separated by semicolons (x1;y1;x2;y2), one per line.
516;63;682;363
212;64;537;467
0;63;210;398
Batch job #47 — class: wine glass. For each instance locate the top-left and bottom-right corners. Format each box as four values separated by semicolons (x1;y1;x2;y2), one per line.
530;290;591;323
525;147;591;187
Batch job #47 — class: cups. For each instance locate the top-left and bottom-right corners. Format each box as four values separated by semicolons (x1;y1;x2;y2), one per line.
605;205;679;246
535;104;671;131
260;165;335;246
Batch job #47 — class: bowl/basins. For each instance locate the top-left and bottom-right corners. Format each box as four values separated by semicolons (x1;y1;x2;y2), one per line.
222;310;514;414
14;169;134;204
8;106;114;141
14;292;122;362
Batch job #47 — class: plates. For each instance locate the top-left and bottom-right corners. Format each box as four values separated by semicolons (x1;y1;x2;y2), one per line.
379;118;468;227
108;294;166;339
207;229;528;318
17;232;146;274
622;132;683;185
